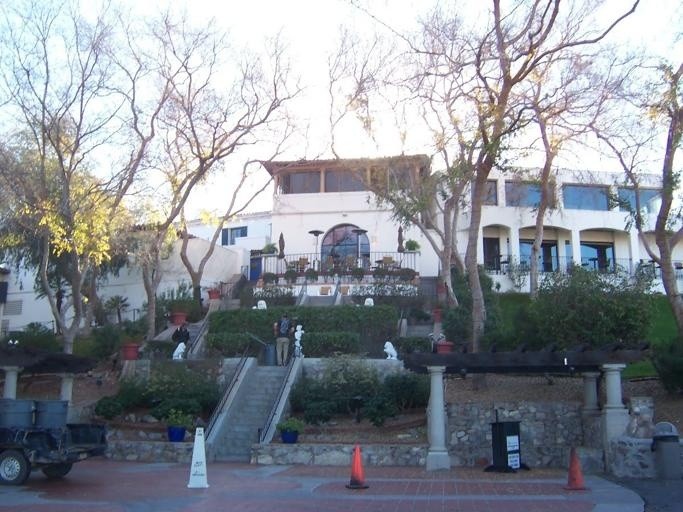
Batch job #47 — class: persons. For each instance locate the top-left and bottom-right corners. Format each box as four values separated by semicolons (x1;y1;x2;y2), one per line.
273;311;294;366
172;322;191;344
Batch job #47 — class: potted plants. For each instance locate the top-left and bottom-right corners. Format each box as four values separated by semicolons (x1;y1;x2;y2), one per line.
162;299;194;325
162;408;192;442
275;417;305;443
204;287;219;298
115;331;142;360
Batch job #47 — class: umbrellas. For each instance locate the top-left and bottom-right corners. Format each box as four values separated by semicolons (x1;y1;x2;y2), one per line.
397;225;409;267
277;232;285;274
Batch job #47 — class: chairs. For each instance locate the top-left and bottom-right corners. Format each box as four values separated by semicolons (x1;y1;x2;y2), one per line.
284;255;403;270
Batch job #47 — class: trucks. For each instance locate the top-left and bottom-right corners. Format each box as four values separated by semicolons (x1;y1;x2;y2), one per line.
0;421;110;485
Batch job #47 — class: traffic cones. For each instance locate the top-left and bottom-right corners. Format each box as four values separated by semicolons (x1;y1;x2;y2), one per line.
344;444;370;490
562;446;587;491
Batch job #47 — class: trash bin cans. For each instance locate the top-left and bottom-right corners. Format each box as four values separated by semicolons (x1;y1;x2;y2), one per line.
262;343;277;366
0;396;35;428
35;399;69;427
483;420;530;473
